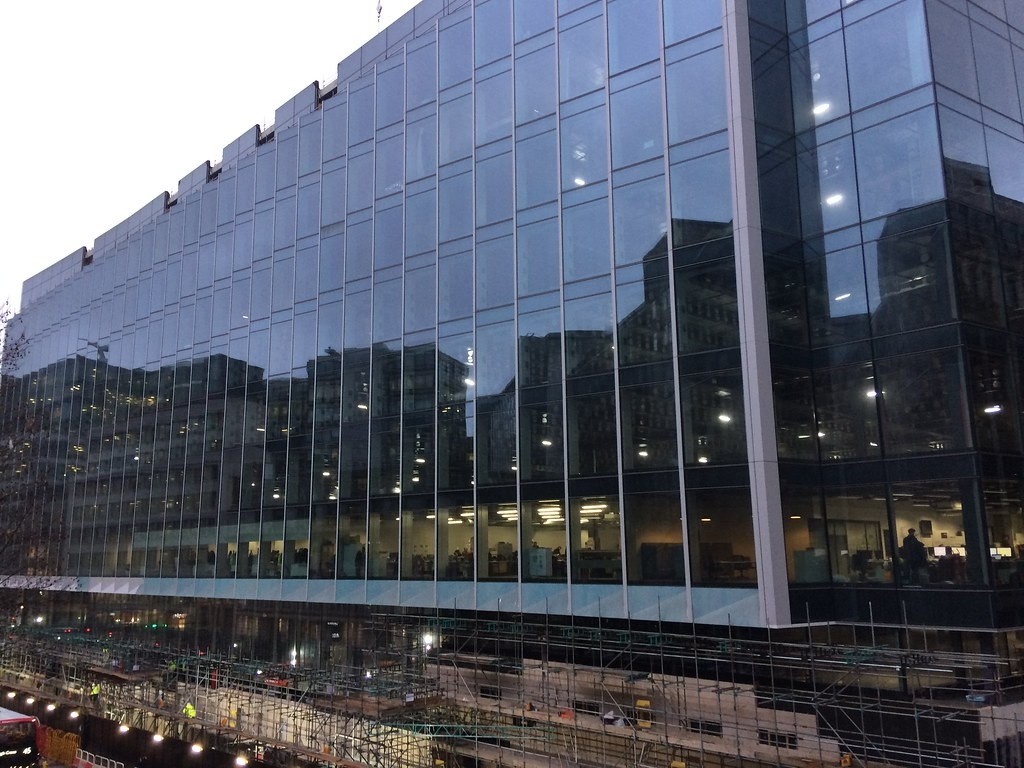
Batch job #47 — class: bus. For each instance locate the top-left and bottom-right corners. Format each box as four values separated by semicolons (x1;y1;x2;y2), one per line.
0;707;41;768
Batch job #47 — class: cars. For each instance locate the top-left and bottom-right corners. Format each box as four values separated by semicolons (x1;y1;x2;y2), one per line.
265;677;288;687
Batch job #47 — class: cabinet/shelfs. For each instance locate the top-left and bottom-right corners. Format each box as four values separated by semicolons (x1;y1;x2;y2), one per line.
794;548;832;583
498;542;510;556
578;549;621;582
699;541;733;578
640;541;682;580
530;548;555;577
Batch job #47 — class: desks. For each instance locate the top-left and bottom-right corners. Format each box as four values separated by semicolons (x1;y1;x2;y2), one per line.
718;559;757;581
921;559;966;583
491;560;513;575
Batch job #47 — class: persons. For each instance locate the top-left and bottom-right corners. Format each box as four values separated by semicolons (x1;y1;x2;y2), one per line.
88;681;100;703
554;545;561;555
182;702;197;719
1000;535;1015;561
533;541;539;549
899;528;927;579
